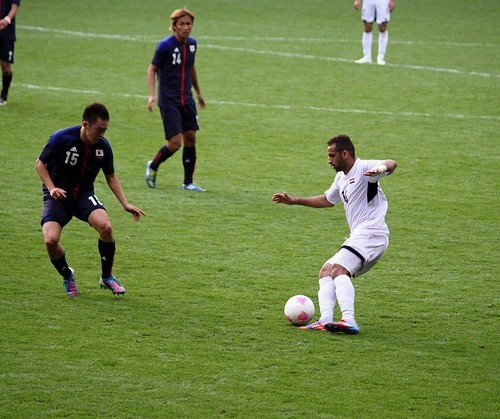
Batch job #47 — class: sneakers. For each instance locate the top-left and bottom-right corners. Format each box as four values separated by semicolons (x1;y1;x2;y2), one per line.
182;183;206;192
60;267;80;297
99;274;126;295
146;160;157;188
301;321;327;331
324;318;361;334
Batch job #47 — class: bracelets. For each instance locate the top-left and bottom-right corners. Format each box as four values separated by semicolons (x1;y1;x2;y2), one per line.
4;16;12;24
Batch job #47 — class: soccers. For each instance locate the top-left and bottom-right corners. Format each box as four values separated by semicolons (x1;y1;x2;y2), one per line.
283;295;315;324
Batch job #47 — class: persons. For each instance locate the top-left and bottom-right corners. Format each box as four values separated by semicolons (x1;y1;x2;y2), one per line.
352;0;396;67
143;7;209;194
0;0;21;106
271;133;399;336
32;102;146;299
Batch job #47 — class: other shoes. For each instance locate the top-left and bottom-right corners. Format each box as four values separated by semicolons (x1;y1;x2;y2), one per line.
0;98;6;105
376;57;387;65
354;56;373;65
